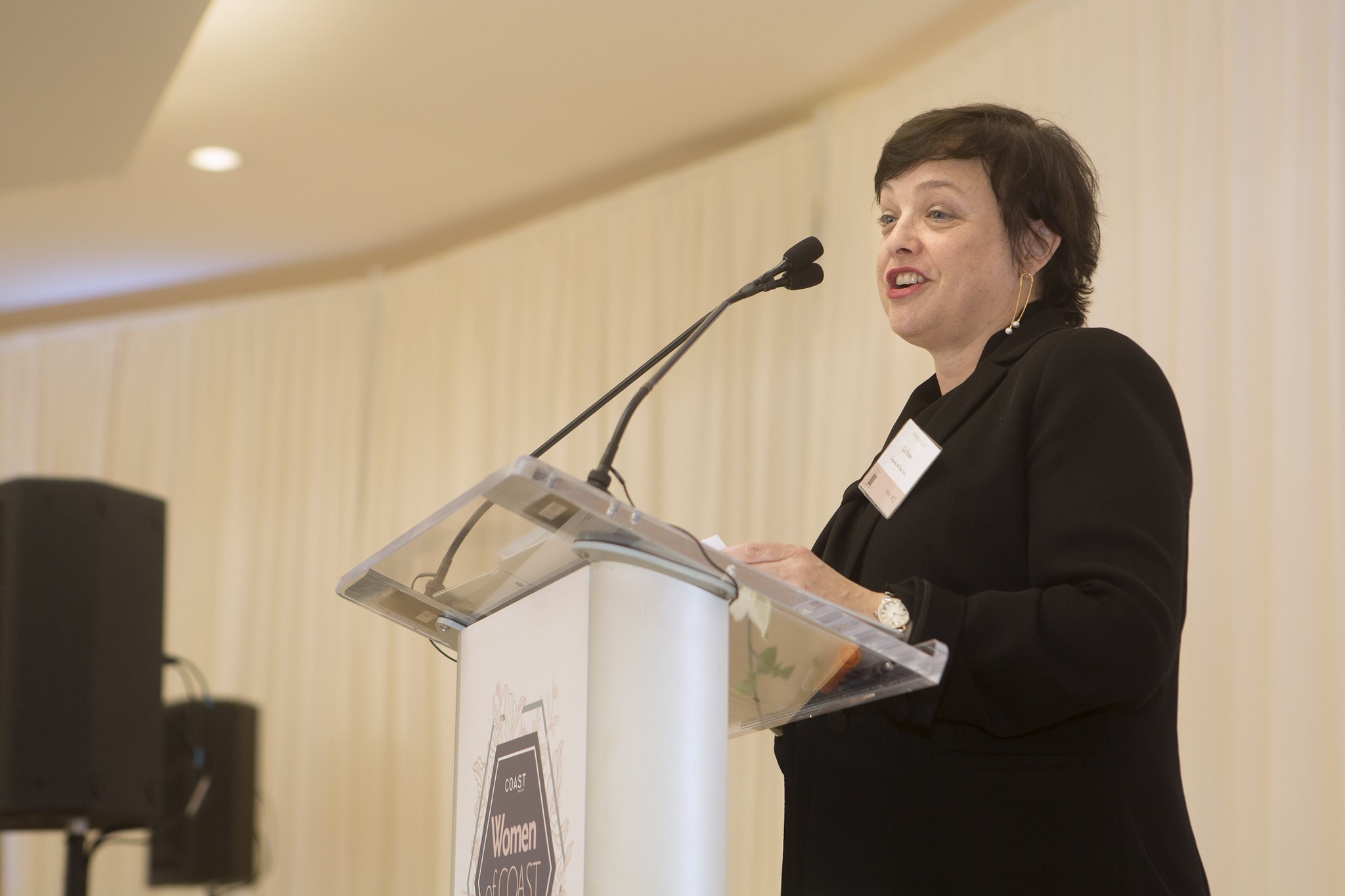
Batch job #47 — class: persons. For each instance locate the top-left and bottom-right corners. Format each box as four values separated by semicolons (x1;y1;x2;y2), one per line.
729;103;1212;896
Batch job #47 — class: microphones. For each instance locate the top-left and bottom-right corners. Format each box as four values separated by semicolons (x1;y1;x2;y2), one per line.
424;236;824;598
586;263;823;498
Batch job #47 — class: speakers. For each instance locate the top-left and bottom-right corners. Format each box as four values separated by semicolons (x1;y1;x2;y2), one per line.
145;698;257;888
0;476;165;834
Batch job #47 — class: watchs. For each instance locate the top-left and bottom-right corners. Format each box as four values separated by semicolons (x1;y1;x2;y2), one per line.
875;590;914;637
